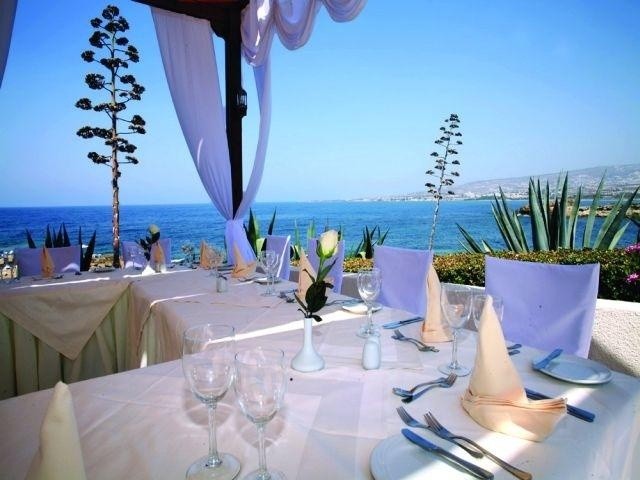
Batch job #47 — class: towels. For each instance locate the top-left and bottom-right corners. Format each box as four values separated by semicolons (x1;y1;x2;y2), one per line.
233;242;256;278
41;246;54;276
22;380;87;480
460;295;567;444
421;263;453;343
298;249;316;302
201;238;216;269
156;241;164;262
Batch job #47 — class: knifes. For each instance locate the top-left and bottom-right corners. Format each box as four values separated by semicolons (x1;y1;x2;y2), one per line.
534;345;564;370
383;315;425;331
402;427;495;480
523;388;595;423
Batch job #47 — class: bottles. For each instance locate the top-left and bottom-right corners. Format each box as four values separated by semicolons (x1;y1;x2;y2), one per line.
154;240;169;273
216;276;227;292
362;333;383;369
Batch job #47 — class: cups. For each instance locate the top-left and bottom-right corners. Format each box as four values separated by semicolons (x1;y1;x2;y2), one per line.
471;294;505;331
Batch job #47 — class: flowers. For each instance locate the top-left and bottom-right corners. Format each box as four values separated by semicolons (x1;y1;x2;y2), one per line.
293;229;340;321
137;224;163;258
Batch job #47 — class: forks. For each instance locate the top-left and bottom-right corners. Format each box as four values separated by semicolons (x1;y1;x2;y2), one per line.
423;411;534;480
395;405;484;460
394;329;440;355
403;374;457;405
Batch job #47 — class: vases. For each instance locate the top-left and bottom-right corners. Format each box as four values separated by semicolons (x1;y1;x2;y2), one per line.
142;261;155;275
291;319;325;372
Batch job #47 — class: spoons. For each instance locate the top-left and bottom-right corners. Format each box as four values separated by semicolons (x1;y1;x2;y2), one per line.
392;375;446;396
389;335;434;352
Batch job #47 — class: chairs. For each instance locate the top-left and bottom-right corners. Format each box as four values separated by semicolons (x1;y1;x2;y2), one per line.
266;235;291;280
374;245;432;318
121;238;170;268
15;245;81;279
481;256;600;363
309;238;344;295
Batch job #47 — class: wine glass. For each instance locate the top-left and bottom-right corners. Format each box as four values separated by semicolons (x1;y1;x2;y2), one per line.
355;268;383;339
437;282;474;377
234;344;290;479
127;245;140;272
181;322;242;479
258;249;282;297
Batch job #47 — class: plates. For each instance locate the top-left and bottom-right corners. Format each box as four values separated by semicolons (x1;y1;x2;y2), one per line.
369;431;492;479
254;276;281;283
340;300;383;315
534;354;613;386
